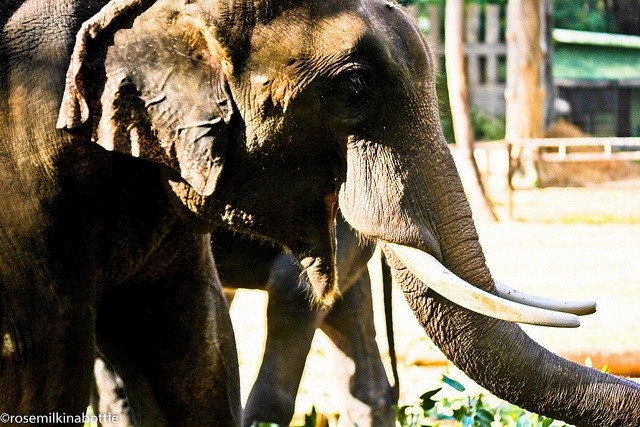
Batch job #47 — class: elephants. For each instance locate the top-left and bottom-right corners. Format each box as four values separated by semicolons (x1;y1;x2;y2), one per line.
0;0;640;426
